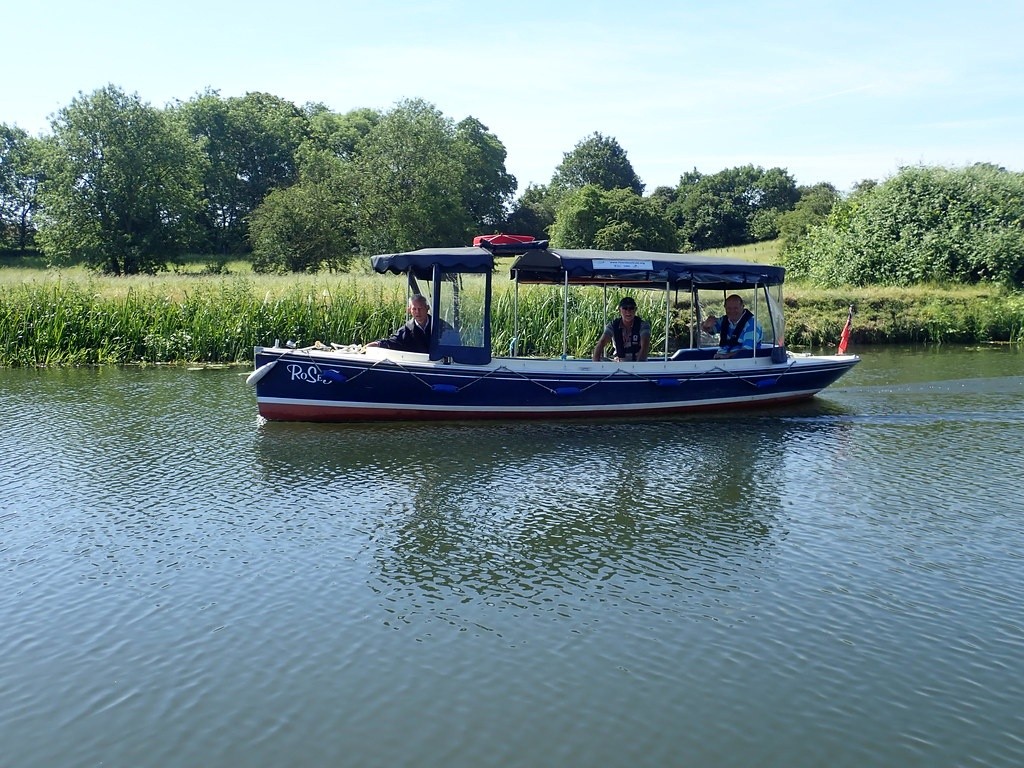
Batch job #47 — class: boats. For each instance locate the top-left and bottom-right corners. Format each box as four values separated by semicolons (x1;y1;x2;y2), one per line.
244;231;864;424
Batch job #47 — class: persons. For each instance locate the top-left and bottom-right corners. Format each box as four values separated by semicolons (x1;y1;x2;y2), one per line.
368;293;459;356
594;297;651;363
700;294;762;361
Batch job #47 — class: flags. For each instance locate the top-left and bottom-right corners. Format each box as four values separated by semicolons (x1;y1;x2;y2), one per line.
839;314;851;352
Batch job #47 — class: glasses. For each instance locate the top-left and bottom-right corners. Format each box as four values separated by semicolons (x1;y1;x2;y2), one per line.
622;307;634;311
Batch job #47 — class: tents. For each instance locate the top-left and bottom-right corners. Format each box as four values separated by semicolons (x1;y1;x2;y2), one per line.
474;234;535;246
